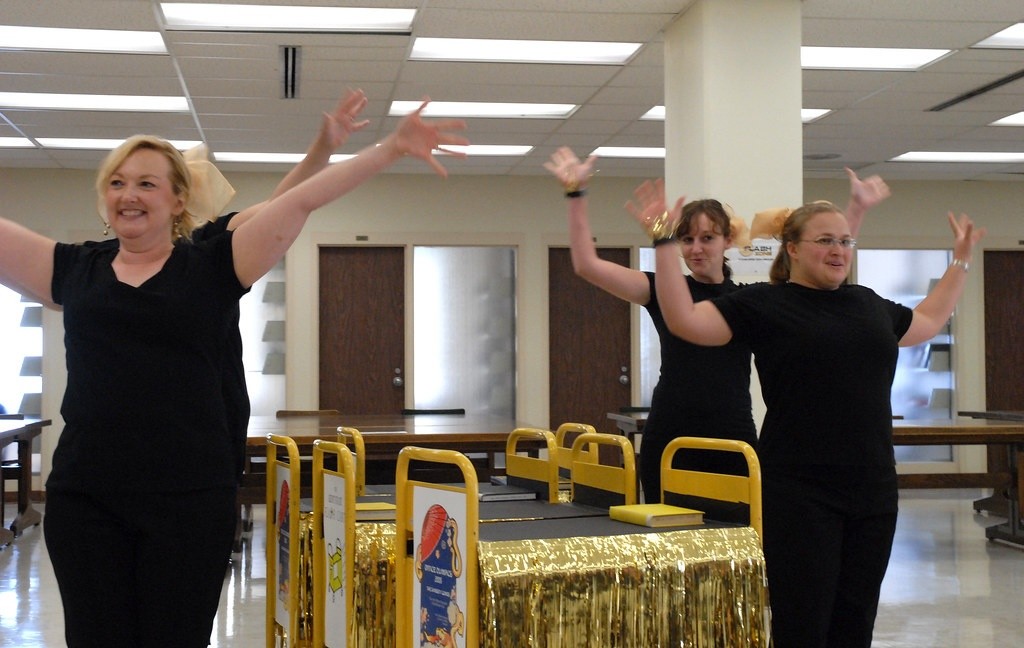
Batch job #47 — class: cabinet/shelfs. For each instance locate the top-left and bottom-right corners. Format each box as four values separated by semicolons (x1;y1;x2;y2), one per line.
266;423;773;648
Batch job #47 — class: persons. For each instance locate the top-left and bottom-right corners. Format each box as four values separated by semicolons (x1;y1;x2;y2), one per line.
542;146;891;525
0;89;469;648
623;179;986;648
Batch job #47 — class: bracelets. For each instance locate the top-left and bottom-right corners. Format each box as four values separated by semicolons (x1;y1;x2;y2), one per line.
951;259;970;272
565;190;587;197
652;236;677;247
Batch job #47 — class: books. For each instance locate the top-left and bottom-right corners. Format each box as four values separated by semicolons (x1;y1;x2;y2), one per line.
478;485;536;502
491;476;572;491
355;502;397;521
609;504;705;529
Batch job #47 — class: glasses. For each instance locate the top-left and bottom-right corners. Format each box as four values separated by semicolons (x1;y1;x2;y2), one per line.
800;236;857;249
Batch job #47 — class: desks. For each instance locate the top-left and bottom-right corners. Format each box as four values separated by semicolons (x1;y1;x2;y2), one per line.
891;410;1024;547
244;413;558;530
0;418;52;550
